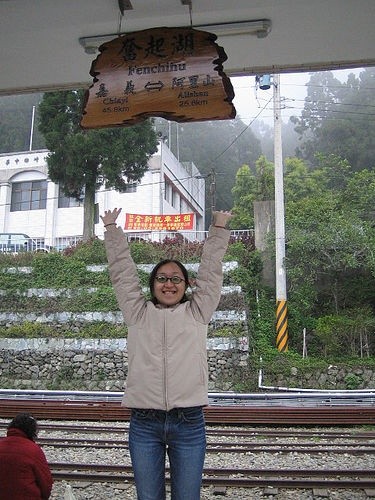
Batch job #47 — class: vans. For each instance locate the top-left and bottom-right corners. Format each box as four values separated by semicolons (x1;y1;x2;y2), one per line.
0;232;60;254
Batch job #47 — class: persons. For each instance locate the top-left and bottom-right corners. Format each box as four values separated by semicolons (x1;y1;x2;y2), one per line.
99;207;238;498
0;413;53;500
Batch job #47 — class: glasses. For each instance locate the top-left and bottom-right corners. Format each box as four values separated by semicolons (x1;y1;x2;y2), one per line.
154;276;186;284
